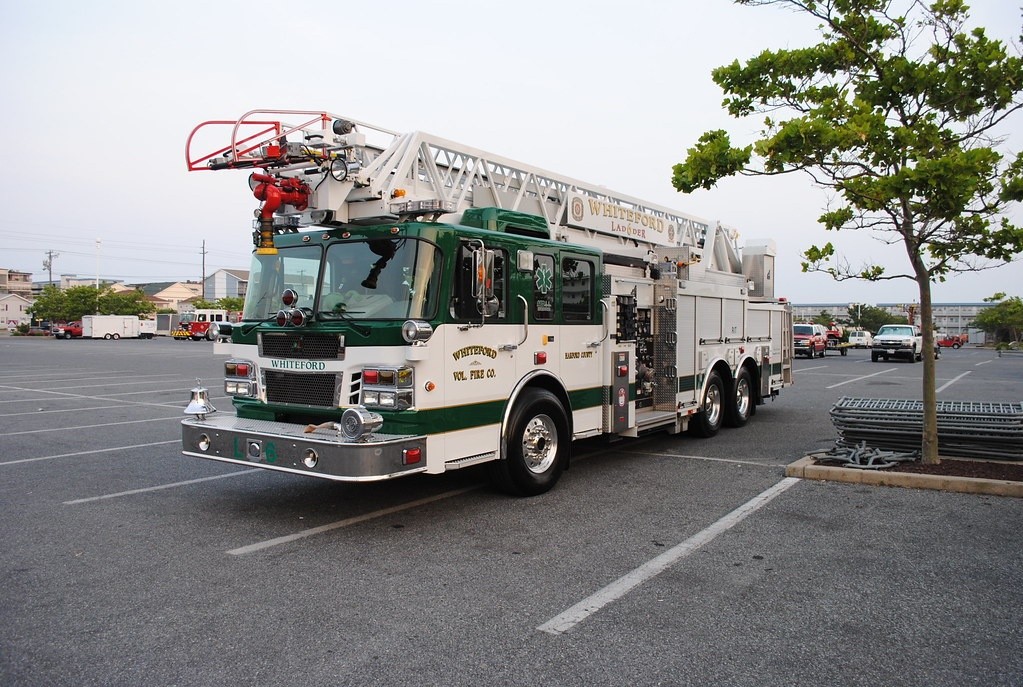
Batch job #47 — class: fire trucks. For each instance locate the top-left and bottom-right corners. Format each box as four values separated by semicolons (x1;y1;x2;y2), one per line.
180;108;795;499
173;308;243;341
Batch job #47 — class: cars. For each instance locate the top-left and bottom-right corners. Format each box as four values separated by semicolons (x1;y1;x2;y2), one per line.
937;335;963;349
850;331;874;349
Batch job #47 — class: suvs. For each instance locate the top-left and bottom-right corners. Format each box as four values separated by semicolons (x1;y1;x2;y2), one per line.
794;322;828;359
872;324;924;363
51;321;82;340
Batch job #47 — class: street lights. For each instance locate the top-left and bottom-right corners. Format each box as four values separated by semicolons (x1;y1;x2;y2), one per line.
96;238;101;311
